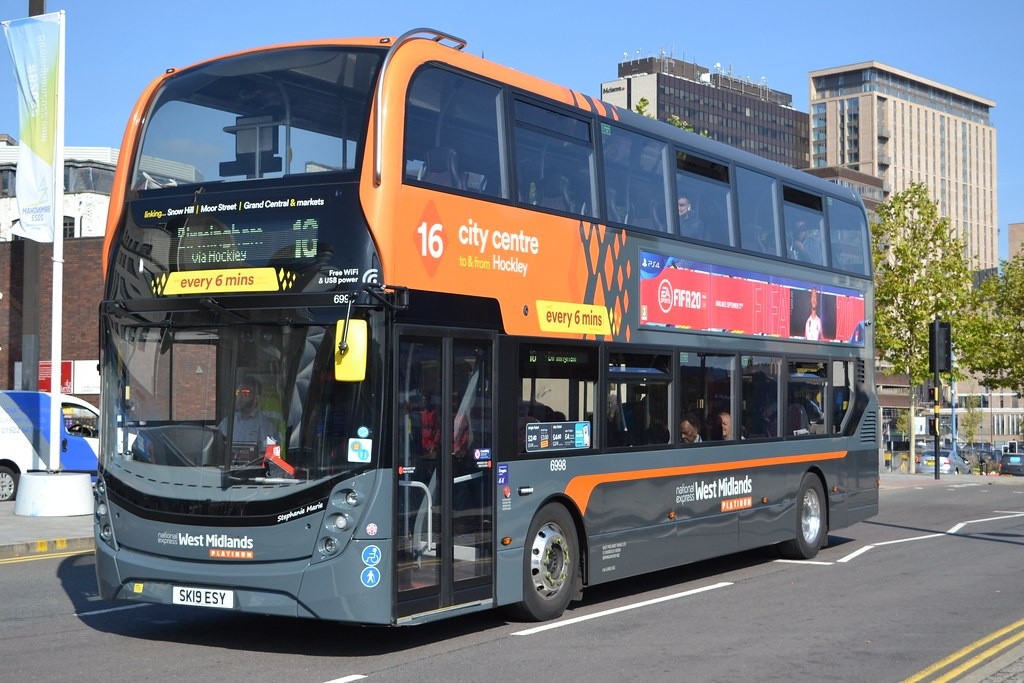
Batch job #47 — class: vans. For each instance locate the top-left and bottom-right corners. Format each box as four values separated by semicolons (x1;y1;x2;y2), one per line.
0;389;138;502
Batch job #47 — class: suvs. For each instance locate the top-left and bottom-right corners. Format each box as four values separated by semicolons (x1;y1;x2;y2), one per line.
999;453;1024;477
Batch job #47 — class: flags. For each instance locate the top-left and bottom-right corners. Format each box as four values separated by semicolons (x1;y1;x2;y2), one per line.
1;10;61;245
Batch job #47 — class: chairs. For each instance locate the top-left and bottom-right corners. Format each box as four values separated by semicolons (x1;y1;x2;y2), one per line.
414;145;666;232
260;410;286;462
787;403;810;436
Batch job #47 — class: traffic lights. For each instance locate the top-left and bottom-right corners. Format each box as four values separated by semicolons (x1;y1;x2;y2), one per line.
1008;441;1017;453
1002;445;1008;455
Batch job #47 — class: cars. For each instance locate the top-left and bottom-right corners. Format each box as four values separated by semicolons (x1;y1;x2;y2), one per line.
915;450;972;477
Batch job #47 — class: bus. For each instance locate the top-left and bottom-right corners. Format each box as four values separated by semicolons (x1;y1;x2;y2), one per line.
93;27;879;632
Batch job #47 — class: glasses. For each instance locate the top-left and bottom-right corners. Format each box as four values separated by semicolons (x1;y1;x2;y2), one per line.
236;389;255;396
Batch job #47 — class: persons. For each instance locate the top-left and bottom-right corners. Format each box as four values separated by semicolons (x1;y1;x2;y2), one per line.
677;195;701;239
804;287;825;342
792;220;822;265
215;375;282;461
448;391;472;456
716;412;746;440
420;392;441;458
680;417;706;443
